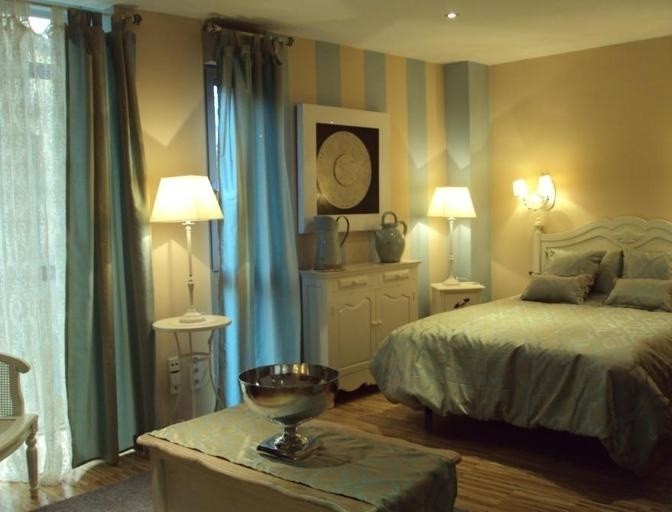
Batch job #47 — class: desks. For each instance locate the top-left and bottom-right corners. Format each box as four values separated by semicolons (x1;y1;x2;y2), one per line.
151;313;234;425
136;402;464;511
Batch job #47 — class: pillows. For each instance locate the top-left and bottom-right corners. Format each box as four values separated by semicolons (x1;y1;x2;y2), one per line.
601;276;671;313
622;243;671;279
520;269;596;306
544;246;626;293
548;248;605;286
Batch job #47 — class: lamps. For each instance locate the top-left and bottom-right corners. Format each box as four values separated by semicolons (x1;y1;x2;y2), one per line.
149;174;225;323
426;186;476;285
511;172;557;211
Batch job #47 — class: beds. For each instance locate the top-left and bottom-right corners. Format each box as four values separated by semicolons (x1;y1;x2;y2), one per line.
367;214;671;475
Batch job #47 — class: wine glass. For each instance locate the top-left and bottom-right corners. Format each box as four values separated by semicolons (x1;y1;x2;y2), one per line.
236;362;340;460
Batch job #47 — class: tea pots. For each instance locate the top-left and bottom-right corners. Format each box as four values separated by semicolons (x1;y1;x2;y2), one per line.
311;212;351;275
374;210;409;264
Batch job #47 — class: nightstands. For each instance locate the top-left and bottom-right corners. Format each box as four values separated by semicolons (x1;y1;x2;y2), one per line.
429;282;484;316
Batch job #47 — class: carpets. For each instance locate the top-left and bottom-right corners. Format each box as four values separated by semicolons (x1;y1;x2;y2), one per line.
26;467;161;511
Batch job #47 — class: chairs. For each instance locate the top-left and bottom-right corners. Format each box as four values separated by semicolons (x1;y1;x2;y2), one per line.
1;351;41;499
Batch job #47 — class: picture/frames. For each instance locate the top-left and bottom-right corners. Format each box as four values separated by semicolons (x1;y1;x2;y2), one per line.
295;104;390;236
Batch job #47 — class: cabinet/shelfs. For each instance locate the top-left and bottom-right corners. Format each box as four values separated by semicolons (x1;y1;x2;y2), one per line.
301;261;423;392
